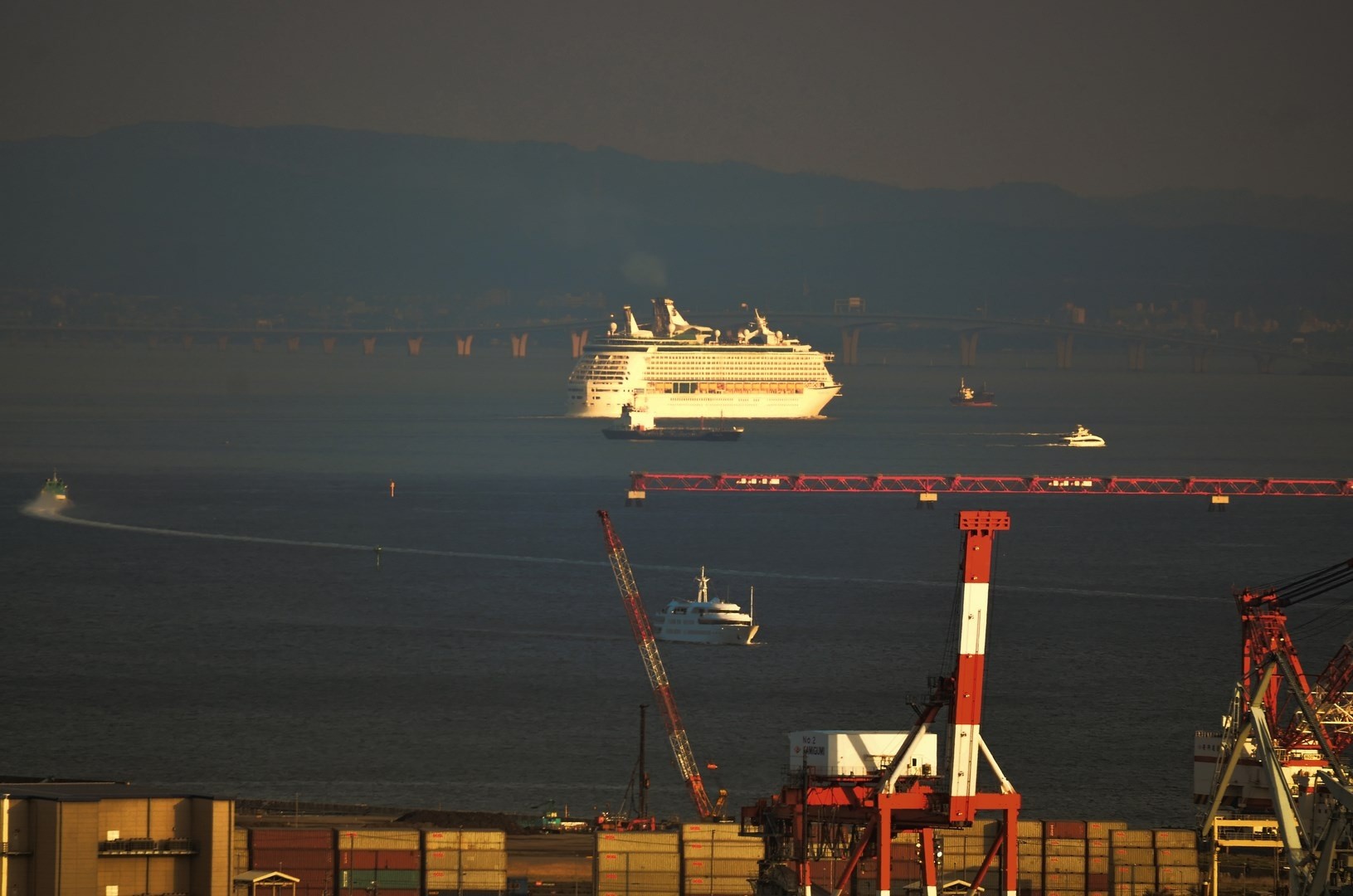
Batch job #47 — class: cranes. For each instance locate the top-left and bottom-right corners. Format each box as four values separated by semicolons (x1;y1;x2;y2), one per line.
628;471;1352;757
597;508;731;821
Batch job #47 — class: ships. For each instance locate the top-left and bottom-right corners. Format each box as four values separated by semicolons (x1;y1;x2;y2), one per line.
565;299;843;419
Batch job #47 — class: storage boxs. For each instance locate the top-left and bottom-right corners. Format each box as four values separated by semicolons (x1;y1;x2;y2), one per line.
232;819;1200;896
100;837;195;850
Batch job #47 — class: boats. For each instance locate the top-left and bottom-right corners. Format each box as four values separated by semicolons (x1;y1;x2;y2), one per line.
1058;424;1105;446
1192;679;1353;820
40;471;73;510
651;566;760;646
949;378;998;408
600;392;745;441
